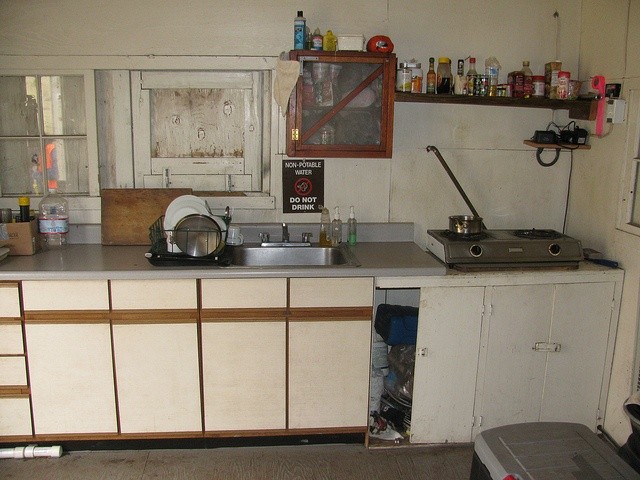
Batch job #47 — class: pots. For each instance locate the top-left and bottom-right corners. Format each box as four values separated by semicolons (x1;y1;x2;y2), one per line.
449;215;483;235
337;34;366;53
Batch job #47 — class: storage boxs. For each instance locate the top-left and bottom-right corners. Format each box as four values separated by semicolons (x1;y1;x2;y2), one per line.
1;215;38;257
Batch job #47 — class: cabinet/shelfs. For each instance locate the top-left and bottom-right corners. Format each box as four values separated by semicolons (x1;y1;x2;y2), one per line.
198;275;373;446
1;280;33;447
475;278;615;442
394;90;590;150
366;275;476;449
17;282;203;451
287;51;396;159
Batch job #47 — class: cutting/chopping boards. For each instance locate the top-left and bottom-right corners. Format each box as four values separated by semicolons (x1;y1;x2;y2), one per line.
102;189;194;247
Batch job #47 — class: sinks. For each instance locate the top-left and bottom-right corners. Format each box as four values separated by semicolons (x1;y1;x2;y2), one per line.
232;246;352;267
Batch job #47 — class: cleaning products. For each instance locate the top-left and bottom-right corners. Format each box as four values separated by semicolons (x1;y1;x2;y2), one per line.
347;205;357;245
331;207;343;247
319;207;332;248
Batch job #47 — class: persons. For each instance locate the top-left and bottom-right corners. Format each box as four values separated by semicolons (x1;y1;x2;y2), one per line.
37;137;59;193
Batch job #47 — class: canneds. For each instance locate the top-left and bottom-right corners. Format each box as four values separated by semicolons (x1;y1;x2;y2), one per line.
477;74;491;96
501;84;506;97
496;84;502;97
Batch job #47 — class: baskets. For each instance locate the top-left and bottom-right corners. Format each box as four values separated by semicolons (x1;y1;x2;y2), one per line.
149;214;231;258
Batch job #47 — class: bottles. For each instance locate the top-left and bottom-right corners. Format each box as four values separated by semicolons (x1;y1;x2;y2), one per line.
37;180;70;252
454;59;466;96
438;58;449;94
322;29;336;51
304;33;314;50
426;57;436;93
313;27;323;51
448;60;452;94
521;61;533;98
467;58;478;96
557;64;570;99
20;198;32;222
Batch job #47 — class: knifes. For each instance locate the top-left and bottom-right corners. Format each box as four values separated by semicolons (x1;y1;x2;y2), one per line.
586;257;617;268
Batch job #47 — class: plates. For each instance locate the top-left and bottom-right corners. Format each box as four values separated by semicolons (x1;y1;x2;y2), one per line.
343;87;376;108
1;245;11;260
382;389;412;428
164;195;226;252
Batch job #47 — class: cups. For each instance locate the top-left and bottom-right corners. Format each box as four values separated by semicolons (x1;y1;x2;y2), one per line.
1;208;12;224
320;127;331;144
226;225;243;246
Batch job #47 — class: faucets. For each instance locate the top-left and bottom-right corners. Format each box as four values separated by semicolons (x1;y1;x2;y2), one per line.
281;221;289;242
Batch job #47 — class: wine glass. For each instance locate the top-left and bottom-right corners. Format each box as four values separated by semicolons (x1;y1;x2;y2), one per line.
18;95;36;137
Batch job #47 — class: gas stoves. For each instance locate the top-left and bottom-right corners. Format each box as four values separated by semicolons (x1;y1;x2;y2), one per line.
425;228;585;269
